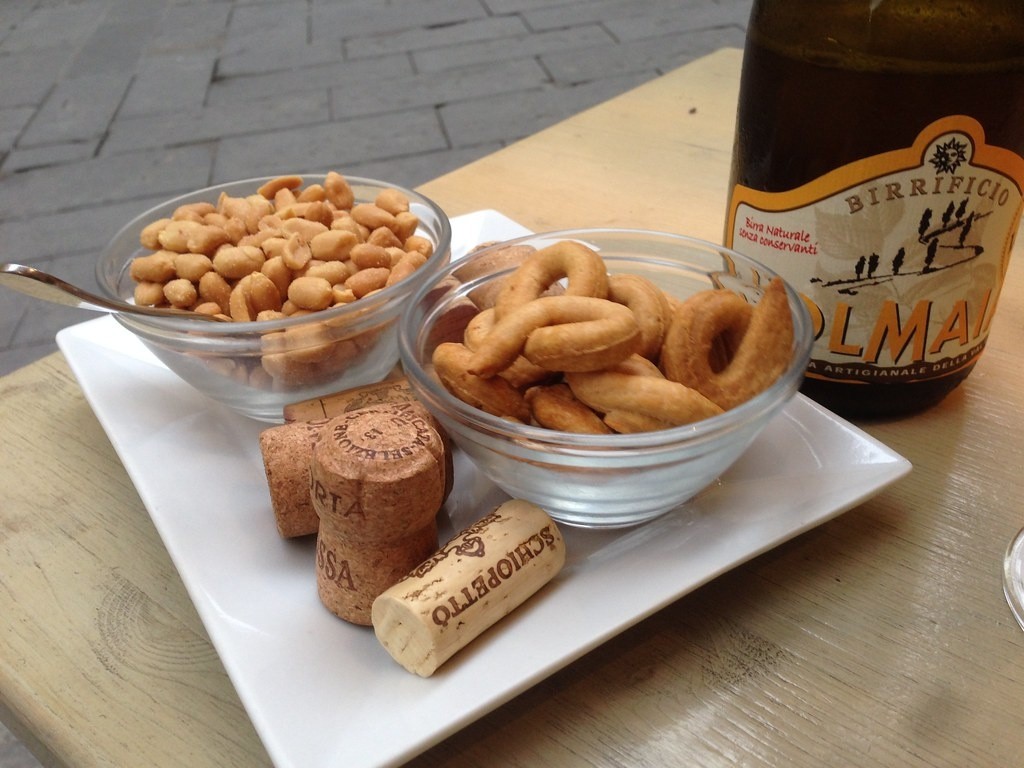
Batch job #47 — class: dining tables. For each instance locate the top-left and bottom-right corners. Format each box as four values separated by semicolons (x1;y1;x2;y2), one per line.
1;42;1022;765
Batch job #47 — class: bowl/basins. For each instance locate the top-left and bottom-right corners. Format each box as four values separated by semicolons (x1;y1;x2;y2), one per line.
94;174;454;424
398;228;813;529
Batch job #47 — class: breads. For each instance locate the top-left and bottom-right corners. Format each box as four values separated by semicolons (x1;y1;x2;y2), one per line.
432;240;799;440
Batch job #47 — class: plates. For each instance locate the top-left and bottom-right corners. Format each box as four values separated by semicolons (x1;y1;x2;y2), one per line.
56;207;915;768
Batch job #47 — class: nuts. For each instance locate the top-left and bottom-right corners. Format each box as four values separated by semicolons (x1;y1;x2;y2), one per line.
129;170;432;388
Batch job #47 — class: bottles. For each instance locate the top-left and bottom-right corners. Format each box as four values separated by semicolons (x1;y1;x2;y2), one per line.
723;0;1024;425
